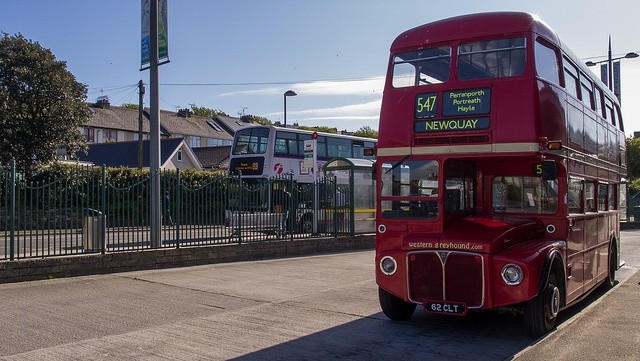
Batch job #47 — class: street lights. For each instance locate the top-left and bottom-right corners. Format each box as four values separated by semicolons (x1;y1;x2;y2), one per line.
284;90;296;128
585;33;640;94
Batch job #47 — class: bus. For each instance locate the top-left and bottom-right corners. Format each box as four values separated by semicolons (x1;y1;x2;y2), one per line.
223;127;378;235
374;9;626;339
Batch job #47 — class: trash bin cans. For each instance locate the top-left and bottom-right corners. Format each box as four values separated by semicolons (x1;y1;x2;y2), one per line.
82;209;106;254
